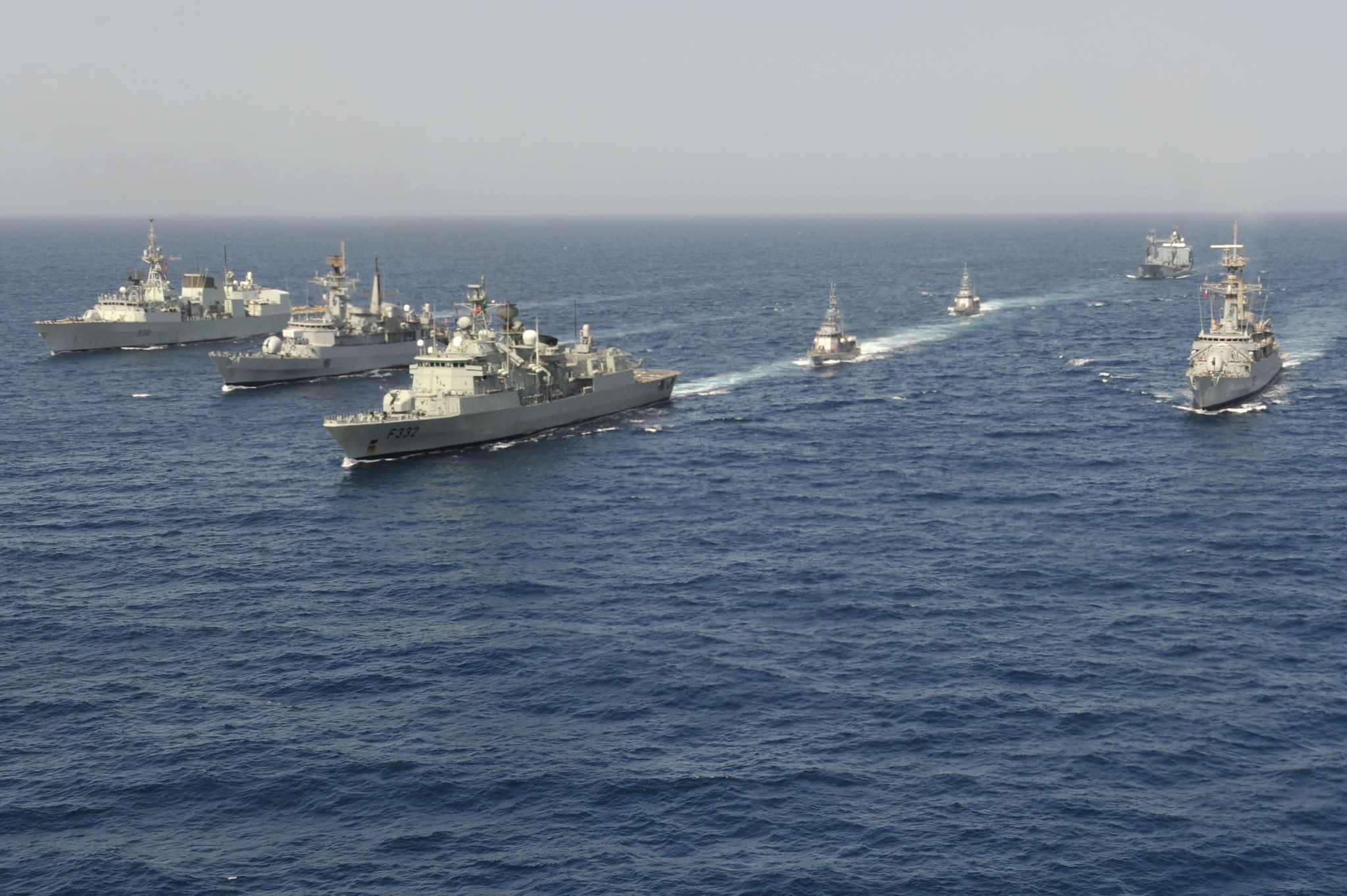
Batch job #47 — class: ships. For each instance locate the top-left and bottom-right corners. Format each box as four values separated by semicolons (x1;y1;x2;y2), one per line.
31;216;295;352
953;261;980;317
208;240;462;385
322;274;680;459
807;282;861;365
1138;225;1196;278
1185;221;1291;408
